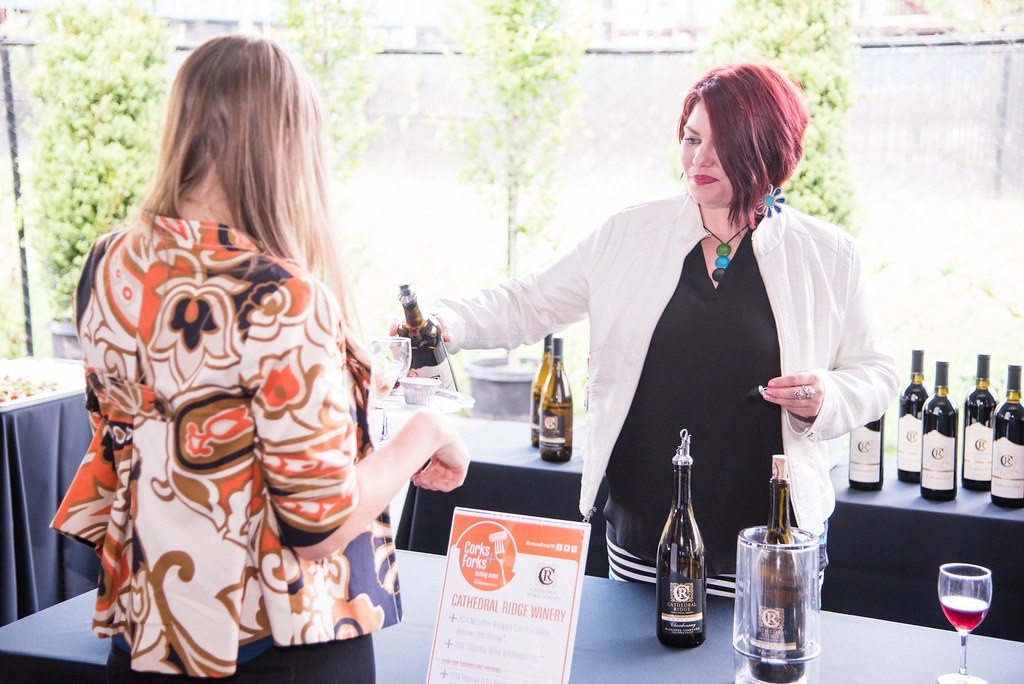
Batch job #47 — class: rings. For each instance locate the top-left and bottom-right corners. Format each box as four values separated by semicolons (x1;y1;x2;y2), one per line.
794;385;815;400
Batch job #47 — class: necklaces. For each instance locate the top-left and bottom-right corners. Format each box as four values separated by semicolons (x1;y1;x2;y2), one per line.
703;221;749;281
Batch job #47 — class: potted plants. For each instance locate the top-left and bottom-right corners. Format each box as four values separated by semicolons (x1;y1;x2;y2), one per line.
441;0;598;419
13;0;178;361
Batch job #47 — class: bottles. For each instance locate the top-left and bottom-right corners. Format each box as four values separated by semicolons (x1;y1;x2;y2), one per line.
848;412;886;491
539;338;572;461
530;334;554;447
920;360;958;499
962;354;996;490
733;454;820;684
655;428;706;648
991;364;1024;507
897;349;929;483
396;284;460;393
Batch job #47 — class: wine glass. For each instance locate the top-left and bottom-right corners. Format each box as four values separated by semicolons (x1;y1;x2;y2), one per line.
371;336;413;443
937;563;993;684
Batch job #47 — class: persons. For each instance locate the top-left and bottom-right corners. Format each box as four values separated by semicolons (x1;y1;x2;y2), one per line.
48;38;470;684
391;63;901;614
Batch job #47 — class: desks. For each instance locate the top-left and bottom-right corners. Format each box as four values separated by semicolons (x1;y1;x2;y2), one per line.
0;549;1024;684
395;419;1024;643
0;355;100;627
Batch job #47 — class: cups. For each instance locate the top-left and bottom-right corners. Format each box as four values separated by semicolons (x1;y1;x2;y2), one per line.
398;376;442;405
